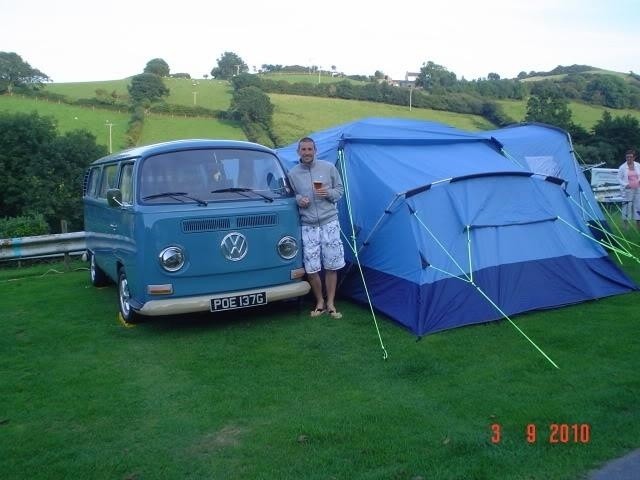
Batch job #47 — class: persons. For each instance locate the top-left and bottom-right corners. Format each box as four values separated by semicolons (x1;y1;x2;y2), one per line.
287;137;346;319
617;149;640;233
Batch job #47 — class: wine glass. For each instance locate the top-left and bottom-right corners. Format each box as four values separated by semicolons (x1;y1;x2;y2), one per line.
313;176;323;203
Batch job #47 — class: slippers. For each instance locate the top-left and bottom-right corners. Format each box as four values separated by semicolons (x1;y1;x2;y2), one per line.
328;311;342;319
311;307;327;316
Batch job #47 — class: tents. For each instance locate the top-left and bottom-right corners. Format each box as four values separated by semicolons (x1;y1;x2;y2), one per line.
475;121;620;252
200;116;639;342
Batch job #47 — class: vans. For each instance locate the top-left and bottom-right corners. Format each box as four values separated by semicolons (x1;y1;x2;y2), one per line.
82;139;312;323
